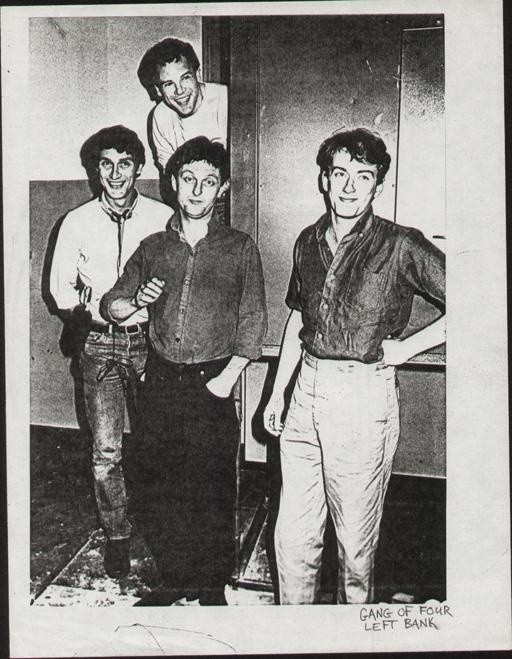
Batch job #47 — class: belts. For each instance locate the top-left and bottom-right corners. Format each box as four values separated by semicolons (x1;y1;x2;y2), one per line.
89;323;150;333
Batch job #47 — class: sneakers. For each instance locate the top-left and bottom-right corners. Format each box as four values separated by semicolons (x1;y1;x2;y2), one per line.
103;537;131;579
132;580;229;606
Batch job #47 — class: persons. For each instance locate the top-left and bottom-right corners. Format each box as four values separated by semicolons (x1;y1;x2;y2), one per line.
96;134;272;607
44;125;184;584
135;34;229;228
262;125;447;603
